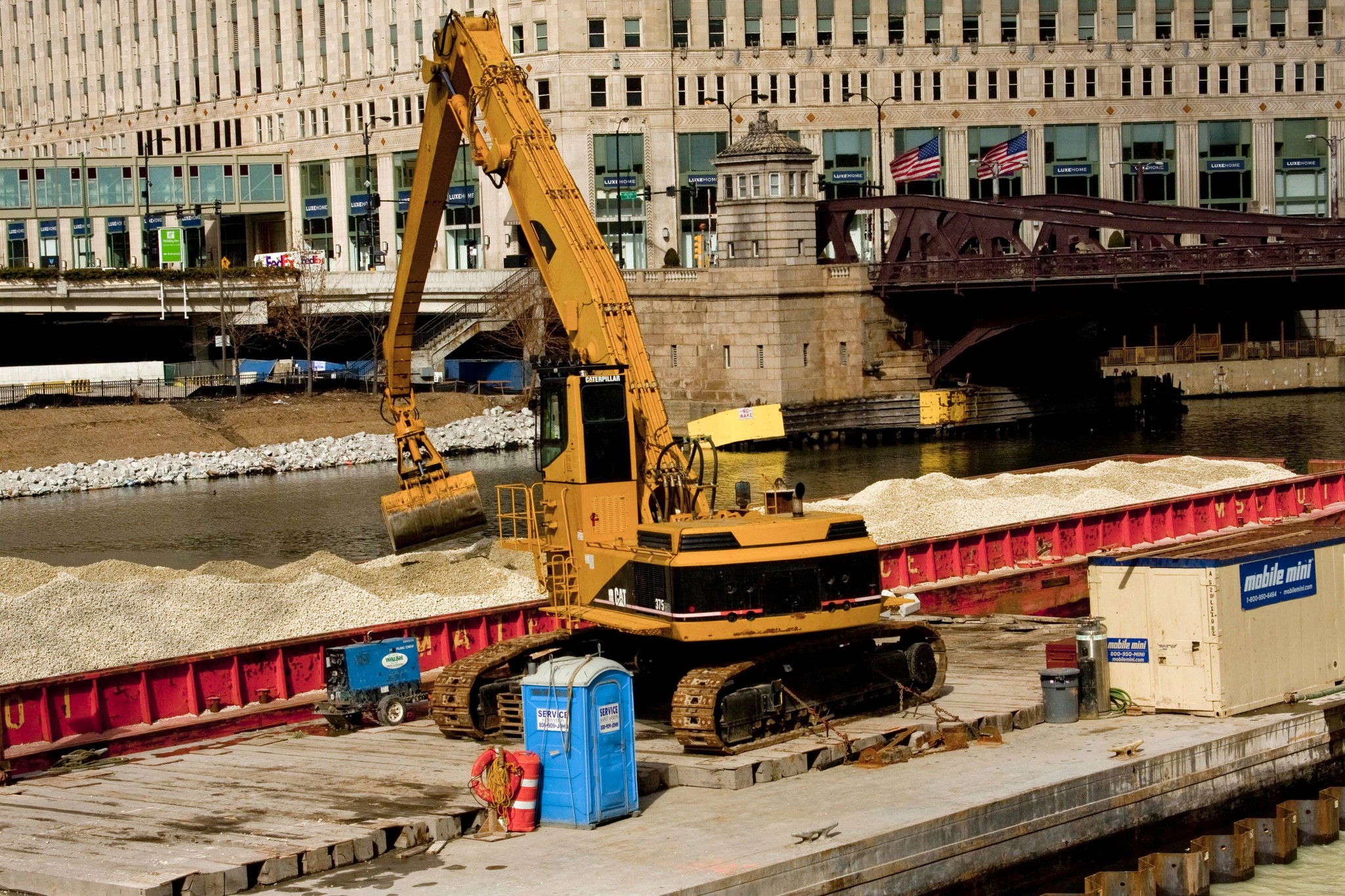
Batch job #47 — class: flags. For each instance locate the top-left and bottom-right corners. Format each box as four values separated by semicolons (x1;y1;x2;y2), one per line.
978;131;1029;180
890;136;941;183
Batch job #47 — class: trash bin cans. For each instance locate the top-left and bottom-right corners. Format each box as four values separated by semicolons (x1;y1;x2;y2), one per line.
1038;668;1080;723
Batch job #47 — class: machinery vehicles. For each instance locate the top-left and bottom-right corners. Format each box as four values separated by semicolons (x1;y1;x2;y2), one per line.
374;8;948;754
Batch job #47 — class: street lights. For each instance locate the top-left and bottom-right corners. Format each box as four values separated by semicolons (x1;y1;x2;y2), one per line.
615;116;629;268
80;146;107;268
361;116;391;272
1304;134;1345;222
144;137;173;268
703;93;768;146
1109;161;1164;247
967;159;1028;205
842;90;903;258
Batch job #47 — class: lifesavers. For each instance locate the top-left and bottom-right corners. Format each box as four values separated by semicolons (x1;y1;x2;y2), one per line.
472;748;522;805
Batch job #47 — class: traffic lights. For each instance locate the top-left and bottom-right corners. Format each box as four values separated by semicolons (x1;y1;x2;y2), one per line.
464;240;471;246
644;184;652;201
688;180;697;197
372;192;381;208
817;173;827;192
372;219;380;236
142;248;150;254
666;186;674;197
215;199;222;215
694;235;702;259
151;231;158;247
176;203;184;219
